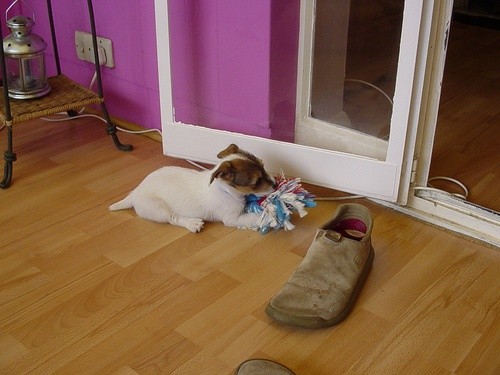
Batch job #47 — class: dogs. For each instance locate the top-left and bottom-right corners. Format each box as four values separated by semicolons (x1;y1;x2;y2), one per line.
109;144;277;233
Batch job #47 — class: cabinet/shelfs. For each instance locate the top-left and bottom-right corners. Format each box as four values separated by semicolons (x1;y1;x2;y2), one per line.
0;1;134;189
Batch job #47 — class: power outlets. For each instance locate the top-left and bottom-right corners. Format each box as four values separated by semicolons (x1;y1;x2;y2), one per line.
75;30;114;68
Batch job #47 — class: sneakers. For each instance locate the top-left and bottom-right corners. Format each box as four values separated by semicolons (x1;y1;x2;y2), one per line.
235;358;297;375
265;202;375;329
